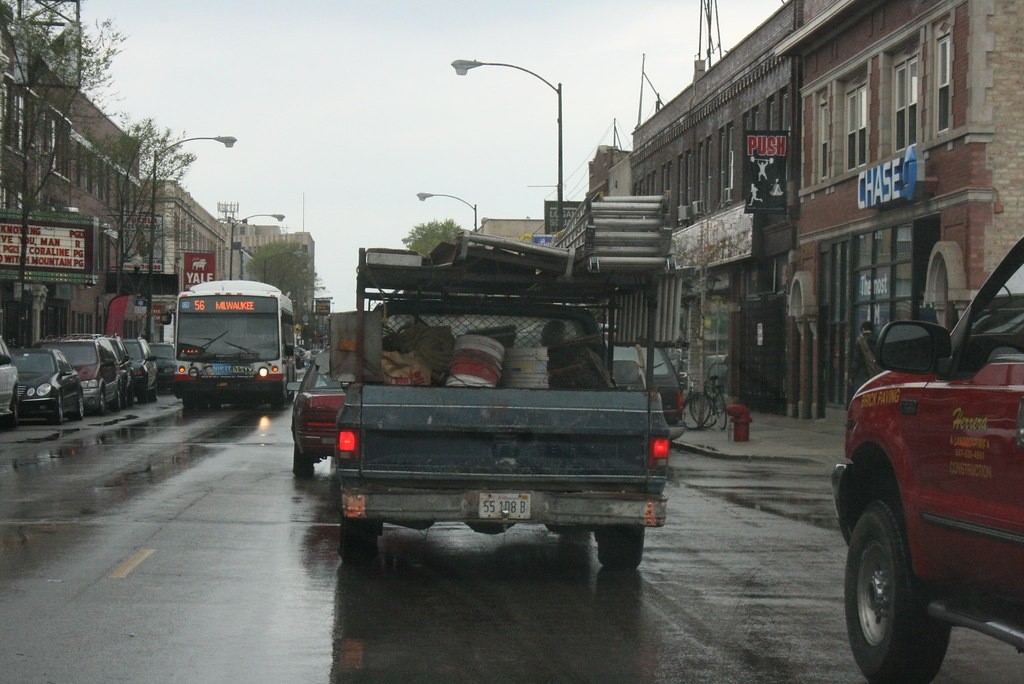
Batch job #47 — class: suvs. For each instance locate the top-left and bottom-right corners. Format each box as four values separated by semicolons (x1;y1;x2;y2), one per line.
30;333;177;416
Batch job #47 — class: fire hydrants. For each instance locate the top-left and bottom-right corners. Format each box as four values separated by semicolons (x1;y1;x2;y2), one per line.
726;405;752;443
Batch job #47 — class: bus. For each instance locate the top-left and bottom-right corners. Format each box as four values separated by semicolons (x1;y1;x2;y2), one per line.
160;280;297;411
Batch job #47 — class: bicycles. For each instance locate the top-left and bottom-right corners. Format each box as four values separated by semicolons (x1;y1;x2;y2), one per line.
680;373;728;430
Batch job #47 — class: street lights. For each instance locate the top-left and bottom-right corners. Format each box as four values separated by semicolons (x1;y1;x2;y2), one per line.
228;214;286;281
452;60;565;234
417;192;478;245
144;136;236;342
263;250;304;283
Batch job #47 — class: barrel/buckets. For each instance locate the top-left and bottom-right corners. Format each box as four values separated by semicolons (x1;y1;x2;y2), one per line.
447;335;549;389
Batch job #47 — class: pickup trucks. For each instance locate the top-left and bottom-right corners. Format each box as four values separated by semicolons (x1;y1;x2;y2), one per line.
334;293;669;573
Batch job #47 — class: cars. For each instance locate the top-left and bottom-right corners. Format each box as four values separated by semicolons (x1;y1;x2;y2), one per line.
596;325;686;441
286;346;348;478
0;333;84;429
286;345;322;370
832;236;1024;684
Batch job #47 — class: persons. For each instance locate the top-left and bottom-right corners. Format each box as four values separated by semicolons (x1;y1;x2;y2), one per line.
851;321;886;396
